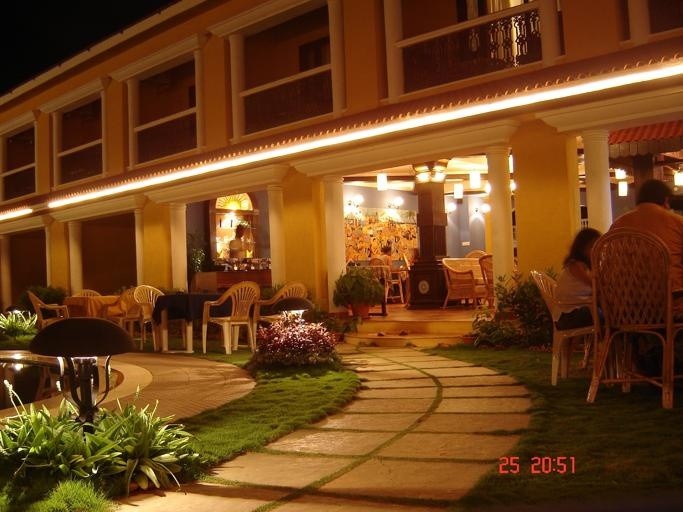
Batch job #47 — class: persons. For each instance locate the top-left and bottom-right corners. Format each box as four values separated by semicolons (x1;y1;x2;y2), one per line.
555;228;654;389
378;246;405;300
610;179;683;397
228;224;251;260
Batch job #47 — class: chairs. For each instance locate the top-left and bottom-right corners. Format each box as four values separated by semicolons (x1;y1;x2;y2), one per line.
26;281;311;355
529;267;619;389
585;228;683;411
348;246;519;312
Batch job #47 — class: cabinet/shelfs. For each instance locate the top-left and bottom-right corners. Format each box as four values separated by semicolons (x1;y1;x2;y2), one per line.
192;270;272;296
206;209;261;262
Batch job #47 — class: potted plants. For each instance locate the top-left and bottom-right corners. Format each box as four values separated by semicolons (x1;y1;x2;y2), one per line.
332;269;387;318
